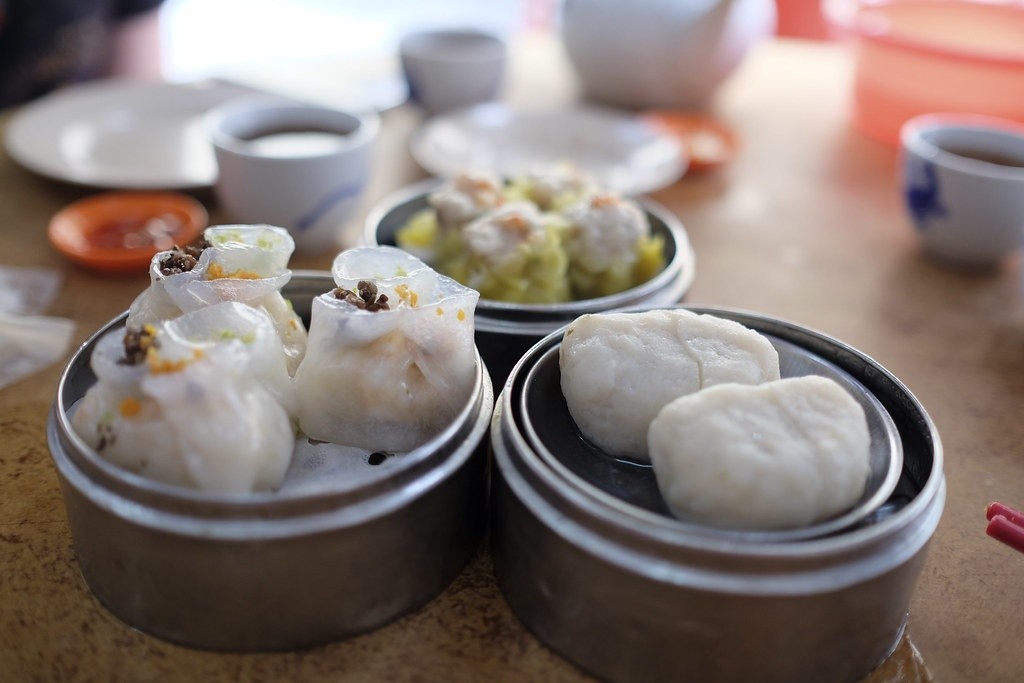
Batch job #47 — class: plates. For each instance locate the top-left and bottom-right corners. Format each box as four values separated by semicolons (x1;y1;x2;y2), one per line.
47;194;207;274
409;101;688;195
1;72;296;186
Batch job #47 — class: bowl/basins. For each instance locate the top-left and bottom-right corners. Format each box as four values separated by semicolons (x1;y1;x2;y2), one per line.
822;0;1024;148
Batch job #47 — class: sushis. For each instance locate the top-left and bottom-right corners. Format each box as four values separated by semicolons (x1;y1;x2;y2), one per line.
403;172;500;259
463;200;568;306
510;175;576;208
567;193;664;295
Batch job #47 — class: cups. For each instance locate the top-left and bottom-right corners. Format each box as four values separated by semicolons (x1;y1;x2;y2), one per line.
209;102;376;259
399;31;505;117
897;123;1024;264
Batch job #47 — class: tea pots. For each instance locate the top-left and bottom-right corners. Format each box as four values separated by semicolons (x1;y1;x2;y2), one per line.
563;0;758;109
